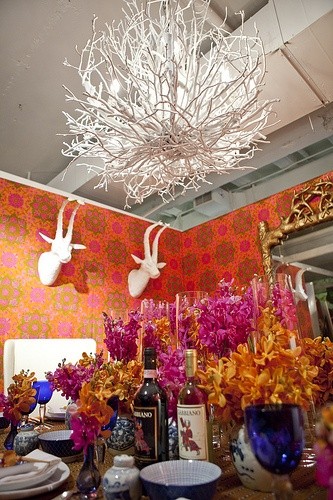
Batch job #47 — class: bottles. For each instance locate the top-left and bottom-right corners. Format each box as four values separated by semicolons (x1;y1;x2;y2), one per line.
133;348;168;470
14;424;38;456
177;349;216;464
101;453;141;500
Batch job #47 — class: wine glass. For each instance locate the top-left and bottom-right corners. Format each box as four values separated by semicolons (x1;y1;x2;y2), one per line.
18;389;40;428
245;403;303;500
33;380;56;430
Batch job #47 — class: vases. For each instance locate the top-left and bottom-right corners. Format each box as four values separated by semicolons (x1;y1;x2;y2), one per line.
228;421;288;493
5;420;19;451
78;432;101;494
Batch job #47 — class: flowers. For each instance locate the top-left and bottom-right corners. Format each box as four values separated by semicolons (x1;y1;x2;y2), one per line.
0;274;333;462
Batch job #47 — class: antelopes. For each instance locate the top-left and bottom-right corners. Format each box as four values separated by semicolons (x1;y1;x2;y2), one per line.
128;219;171;299
38;197;87;285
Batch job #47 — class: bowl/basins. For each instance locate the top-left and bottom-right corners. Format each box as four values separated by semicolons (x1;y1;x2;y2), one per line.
141;460;221;500
38;429;84;462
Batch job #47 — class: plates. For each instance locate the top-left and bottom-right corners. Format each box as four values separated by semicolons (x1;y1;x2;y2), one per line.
44;407;65;421
0;460;70;497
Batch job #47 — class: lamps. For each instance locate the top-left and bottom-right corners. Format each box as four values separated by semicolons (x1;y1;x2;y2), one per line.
53;0;284;212
